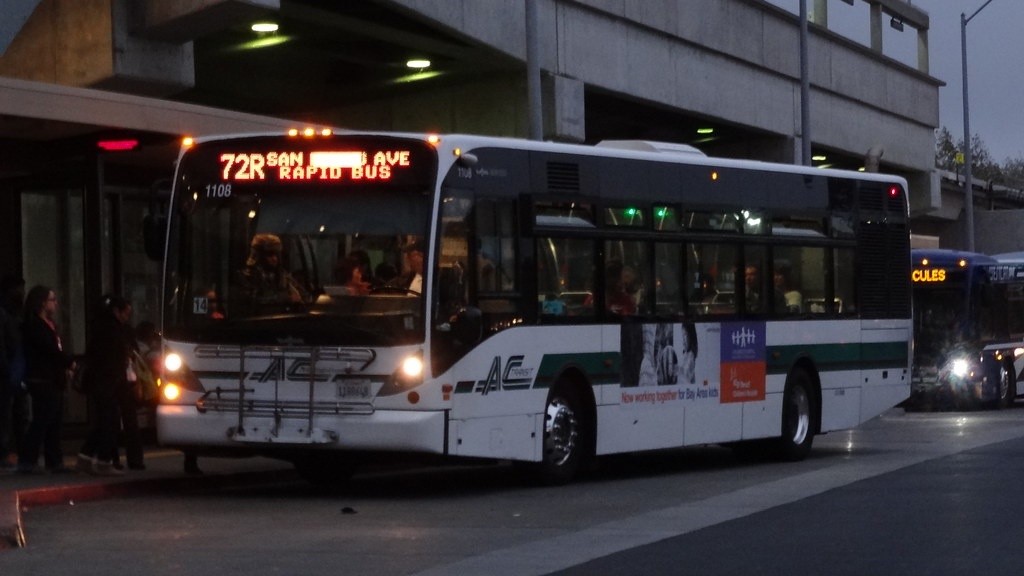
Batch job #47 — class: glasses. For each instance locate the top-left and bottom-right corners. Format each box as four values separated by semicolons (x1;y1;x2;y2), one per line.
264;250;277;257
45;297;56;302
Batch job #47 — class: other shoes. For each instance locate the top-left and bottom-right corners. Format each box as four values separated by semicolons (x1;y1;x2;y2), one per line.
51;462;74;476
112;453;124;470
16;460;44;475
184;461;203;476
83;458;99;475
97;464;124;476
127;460;146;470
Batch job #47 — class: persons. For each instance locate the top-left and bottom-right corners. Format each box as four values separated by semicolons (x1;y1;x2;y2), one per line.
332;234;495;331
227;234;305;313
191;288;225;319
0;274;160;474
539;257;803;316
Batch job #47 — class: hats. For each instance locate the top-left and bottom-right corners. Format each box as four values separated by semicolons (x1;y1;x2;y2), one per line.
246;234;282;270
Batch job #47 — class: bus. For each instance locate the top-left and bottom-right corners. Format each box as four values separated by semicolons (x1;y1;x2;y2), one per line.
152;127;917;488
907;248;1024;410
